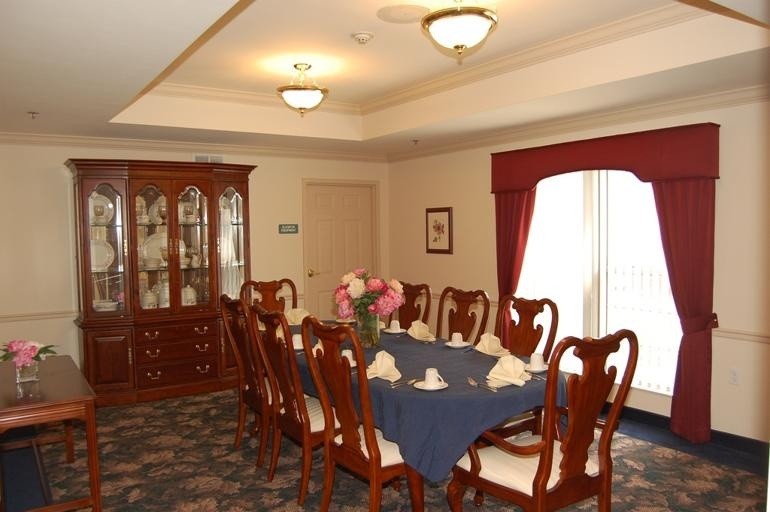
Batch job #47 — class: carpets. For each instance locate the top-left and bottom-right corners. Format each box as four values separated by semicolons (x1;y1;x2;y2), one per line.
29;387;769;511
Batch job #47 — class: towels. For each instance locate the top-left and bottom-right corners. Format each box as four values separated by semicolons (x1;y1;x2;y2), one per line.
406;319;437;342
474;332;512;357
364;351;403;383
486;355;534;389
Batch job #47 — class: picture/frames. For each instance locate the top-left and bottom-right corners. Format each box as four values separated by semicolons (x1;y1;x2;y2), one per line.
424;206;455;255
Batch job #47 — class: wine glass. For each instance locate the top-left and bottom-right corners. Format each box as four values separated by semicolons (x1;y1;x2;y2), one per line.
158;206;168;224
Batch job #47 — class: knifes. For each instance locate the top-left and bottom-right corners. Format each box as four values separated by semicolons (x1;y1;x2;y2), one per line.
390;376;424;386
464;347;474;353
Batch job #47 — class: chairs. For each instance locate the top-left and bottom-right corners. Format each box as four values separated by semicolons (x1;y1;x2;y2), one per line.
299;314;429;511
387;281;433;329
491;294;566;362
447;327;641;512
247;303;346;506
435;285;491;344
238;276;298;434
220;293;310;468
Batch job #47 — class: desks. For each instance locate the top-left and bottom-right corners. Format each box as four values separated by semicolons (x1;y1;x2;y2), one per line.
1;353;102;512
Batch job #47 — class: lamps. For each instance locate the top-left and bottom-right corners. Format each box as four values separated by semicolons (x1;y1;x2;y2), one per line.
276;62;330;120
420;0;499;57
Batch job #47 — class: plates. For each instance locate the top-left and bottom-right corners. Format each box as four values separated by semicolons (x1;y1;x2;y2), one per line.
88;194;114;224
524;363;548;374
178;201;201;223
336;318;356;324
445;342;471;349
143;232;171;267
179;239;186;257
294;345;306;350
383;328;407;334
413;381;449;391
90;240;115;270
350;360;358;368
148;204;163;225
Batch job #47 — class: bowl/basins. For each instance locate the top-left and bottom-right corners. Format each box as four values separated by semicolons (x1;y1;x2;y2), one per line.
93;302;118;311
179;258;191;268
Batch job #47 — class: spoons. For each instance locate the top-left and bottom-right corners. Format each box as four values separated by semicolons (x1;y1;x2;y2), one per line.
392;378;416;390
466;376;497;393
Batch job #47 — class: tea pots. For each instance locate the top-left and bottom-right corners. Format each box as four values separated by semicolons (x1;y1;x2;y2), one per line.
191;254;202;268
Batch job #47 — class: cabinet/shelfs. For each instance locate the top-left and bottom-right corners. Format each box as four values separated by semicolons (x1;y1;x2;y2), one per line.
62;157;258;407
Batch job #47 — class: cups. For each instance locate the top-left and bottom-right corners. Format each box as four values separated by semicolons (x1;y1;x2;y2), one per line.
341;349;353;362
529;353;544;370
151;214;162;225
451;332;462;345
95;216;106;226
136;216;154;224
390;320;401;332
143;258;162;270
186;214;196;224
292;334;303;346
424;367;445;386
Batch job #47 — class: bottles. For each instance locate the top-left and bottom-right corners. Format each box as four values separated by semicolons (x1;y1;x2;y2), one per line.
142;280;197;309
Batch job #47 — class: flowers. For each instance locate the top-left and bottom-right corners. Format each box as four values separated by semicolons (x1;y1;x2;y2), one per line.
329;267;407;320
0;337;58;367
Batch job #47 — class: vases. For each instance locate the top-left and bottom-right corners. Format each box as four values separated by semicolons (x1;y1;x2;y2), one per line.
14;358;41;384
357;312;382;349
